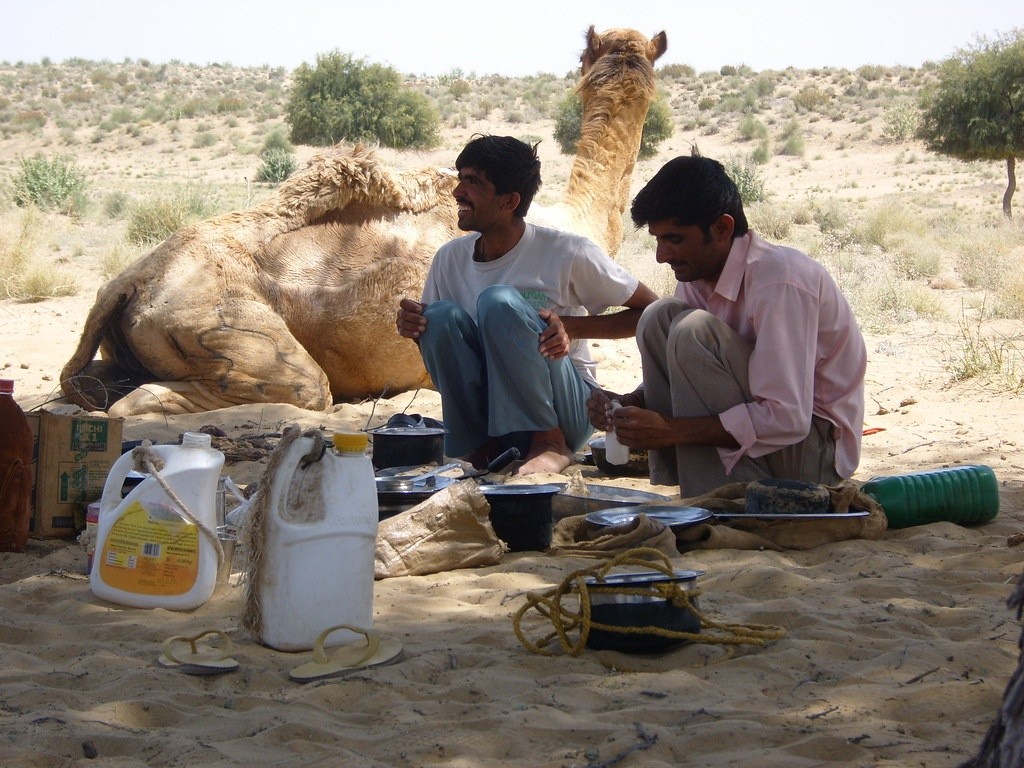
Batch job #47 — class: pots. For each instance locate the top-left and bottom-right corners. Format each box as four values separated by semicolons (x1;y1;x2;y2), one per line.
475;482;562;552
370;425;449;469
584;570;704;656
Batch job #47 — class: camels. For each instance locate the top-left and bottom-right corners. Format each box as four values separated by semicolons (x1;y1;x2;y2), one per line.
59;24;667;419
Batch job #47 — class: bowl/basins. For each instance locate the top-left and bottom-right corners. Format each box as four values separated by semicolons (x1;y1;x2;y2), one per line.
588;437;649;475
385;413;444;428
375;479;414;492
550;483;673;519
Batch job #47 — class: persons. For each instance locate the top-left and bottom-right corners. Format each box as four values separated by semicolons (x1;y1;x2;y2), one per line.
396;134;660;479
585;154;868;500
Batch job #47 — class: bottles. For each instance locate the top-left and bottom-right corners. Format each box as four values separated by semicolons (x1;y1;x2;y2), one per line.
861;464;1000;528
0;379;34;555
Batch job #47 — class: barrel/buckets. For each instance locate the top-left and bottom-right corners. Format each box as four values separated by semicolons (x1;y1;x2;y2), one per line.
86;429;226;611
249;428;379;651
